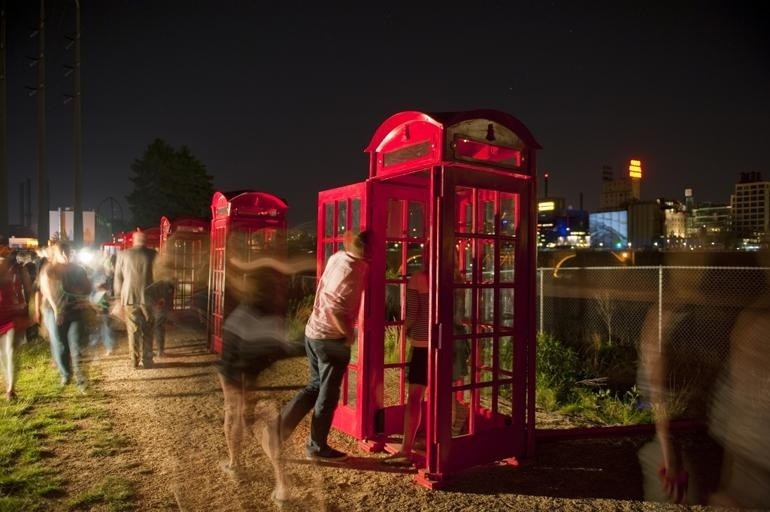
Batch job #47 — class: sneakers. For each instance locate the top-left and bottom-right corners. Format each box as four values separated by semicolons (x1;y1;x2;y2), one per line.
62;371;73;385
79;384;92;397
7;391;18;402
308;445;348;462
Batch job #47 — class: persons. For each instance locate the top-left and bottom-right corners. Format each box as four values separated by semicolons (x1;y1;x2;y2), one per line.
380;233;471;463
1;226;180;409
276;227;371;460
624;220;770;510
215;248;298;507
